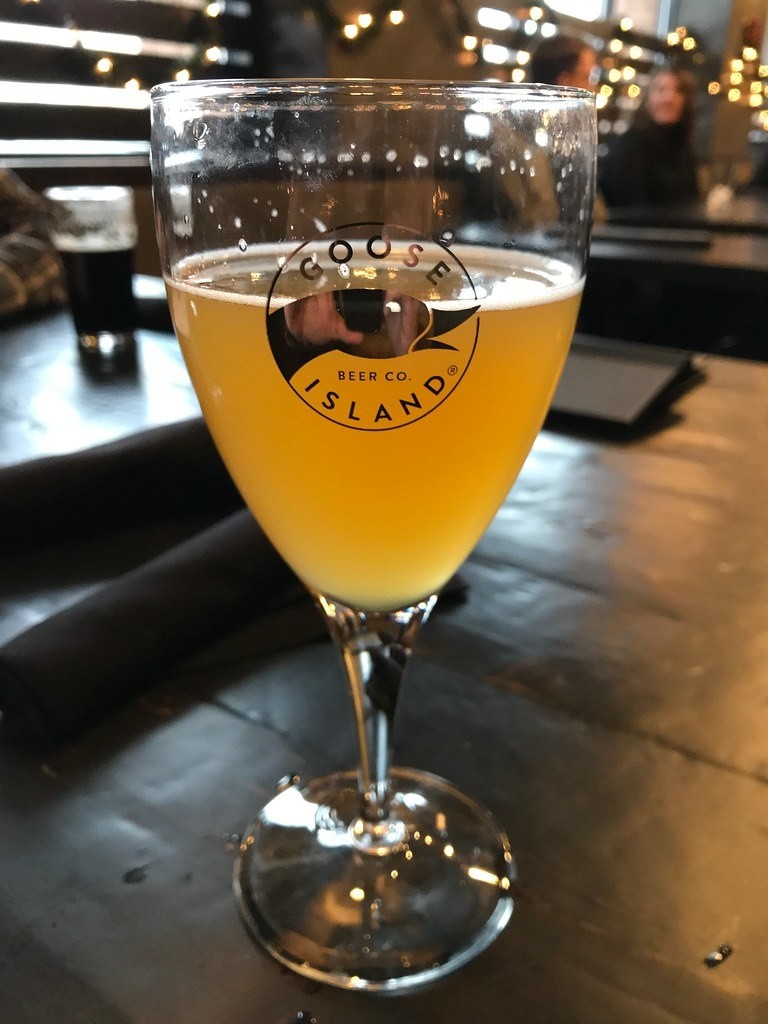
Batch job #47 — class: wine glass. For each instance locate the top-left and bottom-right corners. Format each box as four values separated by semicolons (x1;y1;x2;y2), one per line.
133;68;594;1005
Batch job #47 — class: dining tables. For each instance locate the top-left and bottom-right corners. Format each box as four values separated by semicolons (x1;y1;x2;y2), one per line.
454;214;768;274
0;275;768;1024
609;195;767;231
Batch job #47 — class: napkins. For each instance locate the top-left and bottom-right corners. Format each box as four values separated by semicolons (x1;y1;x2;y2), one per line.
706;181;737;223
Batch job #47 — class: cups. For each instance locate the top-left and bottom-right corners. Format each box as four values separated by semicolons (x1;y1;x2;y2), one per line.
50;205;136;356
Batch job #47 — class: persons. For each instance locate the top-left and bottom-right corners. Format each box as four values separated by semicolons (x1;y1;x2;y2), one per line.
594;63;701;208
489;32;609;236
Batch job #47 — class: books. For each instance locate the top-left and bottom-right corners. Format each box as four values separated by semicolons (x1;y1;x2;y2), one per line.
550;331;702;436
